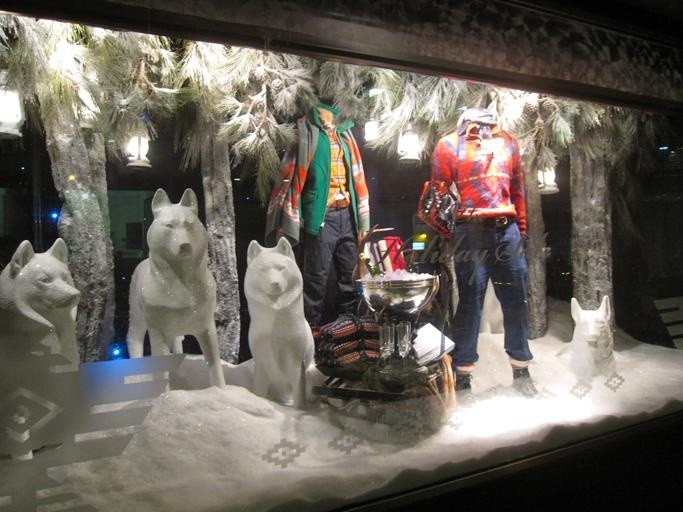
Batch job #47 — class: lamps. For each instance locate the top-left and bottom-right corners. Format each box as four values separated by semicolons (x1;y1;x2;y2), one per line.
396;120;424;164
536;163;559;196
0;66;27;143
120;122;152;170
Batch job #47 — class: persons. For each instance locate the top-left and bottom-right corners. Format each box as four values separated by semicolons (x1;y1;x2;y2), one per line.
417;109;538;398
264;99;370;331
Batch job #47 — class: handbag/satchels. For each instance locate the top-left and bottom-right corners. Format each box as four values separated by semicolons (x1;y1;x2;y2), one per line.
417;180;460;241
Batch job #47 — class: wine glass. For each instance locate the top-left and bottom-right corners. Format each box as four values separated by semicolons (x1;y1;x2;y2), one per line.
378;320;414;372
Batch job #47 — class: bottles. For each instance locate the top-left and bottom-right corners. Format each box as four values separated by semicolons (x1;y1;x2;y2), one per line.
363;258;383;277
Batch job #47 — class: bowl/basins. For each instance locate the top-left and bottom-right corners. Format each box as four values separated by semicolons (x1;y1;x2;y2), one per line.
359;277;438;315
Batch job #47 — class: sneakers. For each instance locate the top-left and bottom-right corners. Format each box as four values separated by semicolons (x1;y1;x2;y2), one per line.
511;365;539;398
454;371;477;409
